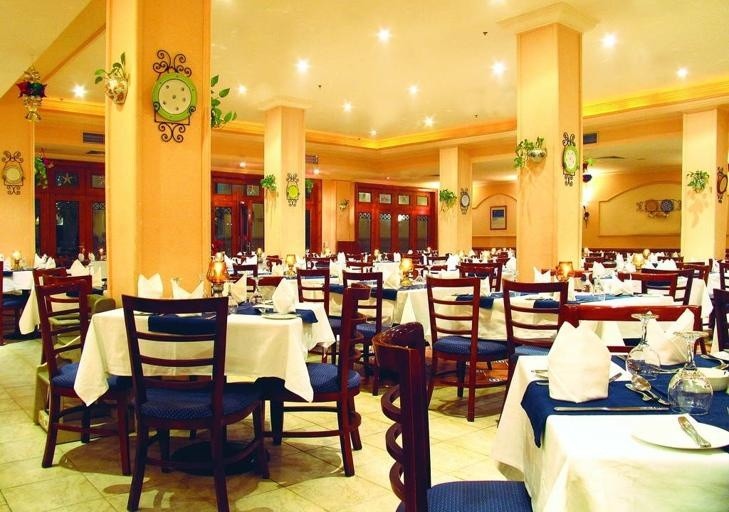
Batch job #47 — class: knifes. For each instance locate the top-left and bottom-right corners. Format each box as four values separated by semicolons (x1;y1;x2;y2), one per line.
608;372;622;384
553;406;670;412
677;416;709;448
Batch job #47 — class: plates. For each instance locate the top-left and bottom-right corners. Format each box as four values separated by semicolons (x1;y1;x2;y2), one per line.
635;421;728;450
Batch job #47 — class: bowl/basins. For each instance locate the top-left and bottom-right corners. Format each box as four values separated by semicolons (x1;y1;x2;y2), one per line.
698;365;729;392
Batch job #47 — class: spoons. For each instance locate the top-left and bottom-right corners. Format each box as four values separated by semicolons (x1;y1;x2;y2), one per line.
632;372;668;406
625;383;652;402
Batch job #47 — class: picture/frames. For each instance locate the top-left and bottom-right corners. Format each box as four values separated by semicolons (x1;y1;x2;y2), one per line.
490;205;507;230
379;193;392;204
416;195;428;207
397;194;410;205
358;192;372;203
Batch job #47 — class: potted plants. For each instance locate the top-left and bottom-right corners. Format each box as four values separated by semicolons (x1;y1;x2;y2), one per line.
94;50;127;104
513;135;546;169
686;169;709;193
260;174;276;198
305;178;314;199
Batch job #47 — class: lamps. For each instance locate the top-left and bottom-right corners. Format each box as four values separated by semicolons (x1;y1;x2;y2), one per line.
15;62;47;122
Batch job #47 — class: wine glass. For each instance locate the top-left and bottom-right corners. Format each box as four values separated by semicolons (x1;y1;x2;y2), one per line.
668;331;715;415
457;248;719;303
240;250;322;278
125;282;301;321
626;314;661;378
363;253;434;287
